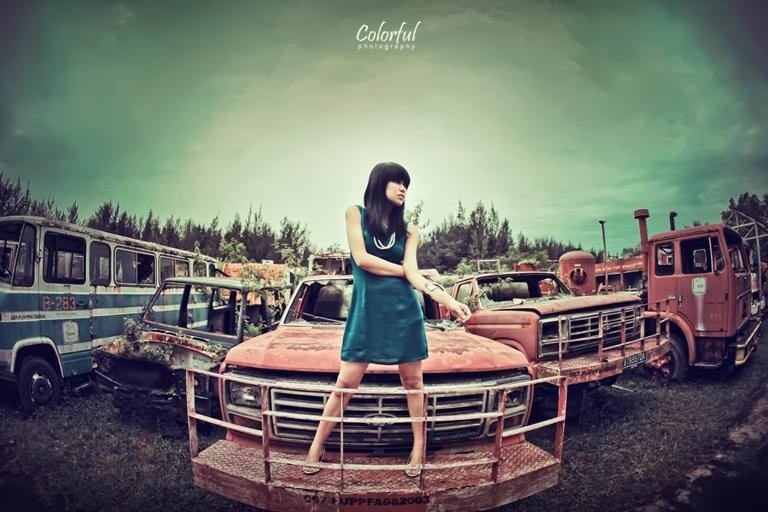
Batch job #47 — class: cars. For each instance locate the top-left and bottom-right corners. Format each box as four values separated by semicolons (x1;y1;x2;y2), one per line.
91;276;302;430
178;272;569;510
429;270;673;422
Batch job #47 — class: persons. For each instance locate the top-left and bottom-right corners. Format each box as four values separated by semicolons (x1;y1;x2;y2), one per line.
302;160;473;478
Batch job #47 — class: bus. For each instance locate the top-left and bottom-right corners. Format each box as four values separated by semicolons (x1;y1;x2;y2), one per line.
0;214;226;411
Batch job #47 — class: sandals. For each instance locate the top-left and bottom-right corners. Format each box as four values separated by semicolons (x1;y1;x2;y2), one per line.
406;457;422;476
302;446;325;474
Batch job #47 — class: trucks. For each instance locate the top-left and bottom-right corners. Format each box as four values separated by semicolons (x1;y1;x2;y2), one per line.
512;209;762;384
